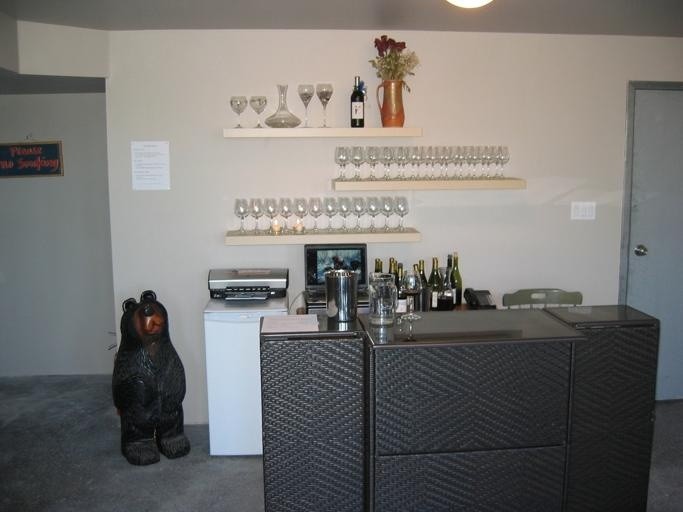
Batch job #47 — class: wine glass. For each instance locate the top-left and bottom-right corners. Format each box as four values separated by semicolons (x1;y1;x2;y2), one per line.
316;83;333;127
234;196;409;234
230;96;247;129
250;96;267;128
298;84;315;127
399;269;422;319
334;146;510;180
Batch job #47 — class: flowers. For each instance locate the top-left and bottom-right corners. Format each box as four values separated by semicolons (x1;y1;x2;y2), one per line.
370;35;419;92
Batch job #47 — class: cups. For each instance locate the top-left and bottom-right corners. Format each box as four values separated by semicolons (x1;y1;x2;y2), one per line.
367;273;398;326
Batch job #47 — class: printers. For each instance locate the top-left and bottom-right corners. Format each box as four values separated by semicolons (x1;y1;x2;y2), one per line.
205;267;290;303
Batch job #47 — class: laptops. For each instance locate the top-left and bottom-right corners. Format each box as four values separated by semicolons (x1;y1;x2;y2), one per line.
303;243;370;306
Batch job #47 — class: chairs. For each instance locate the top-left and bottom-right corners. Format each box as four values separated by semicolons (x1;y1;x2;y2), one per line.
503;287;582;310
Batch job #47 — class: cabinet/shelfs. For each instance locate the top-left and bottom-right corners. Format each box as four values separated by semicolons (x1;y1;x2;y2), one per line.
259;305;661;512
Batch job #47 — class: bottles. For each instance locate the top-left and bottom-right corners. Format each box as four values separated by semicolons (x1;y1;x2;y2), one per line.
350;77;364;128
375;251;462;314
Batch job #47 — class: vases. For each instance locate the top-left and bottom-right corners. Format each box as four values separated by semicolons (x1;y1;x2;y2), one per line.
375;80;406;127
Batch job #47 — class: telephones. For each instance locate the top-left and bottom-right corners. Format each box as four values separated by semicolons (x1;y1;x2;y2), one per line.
463;288;496;310
